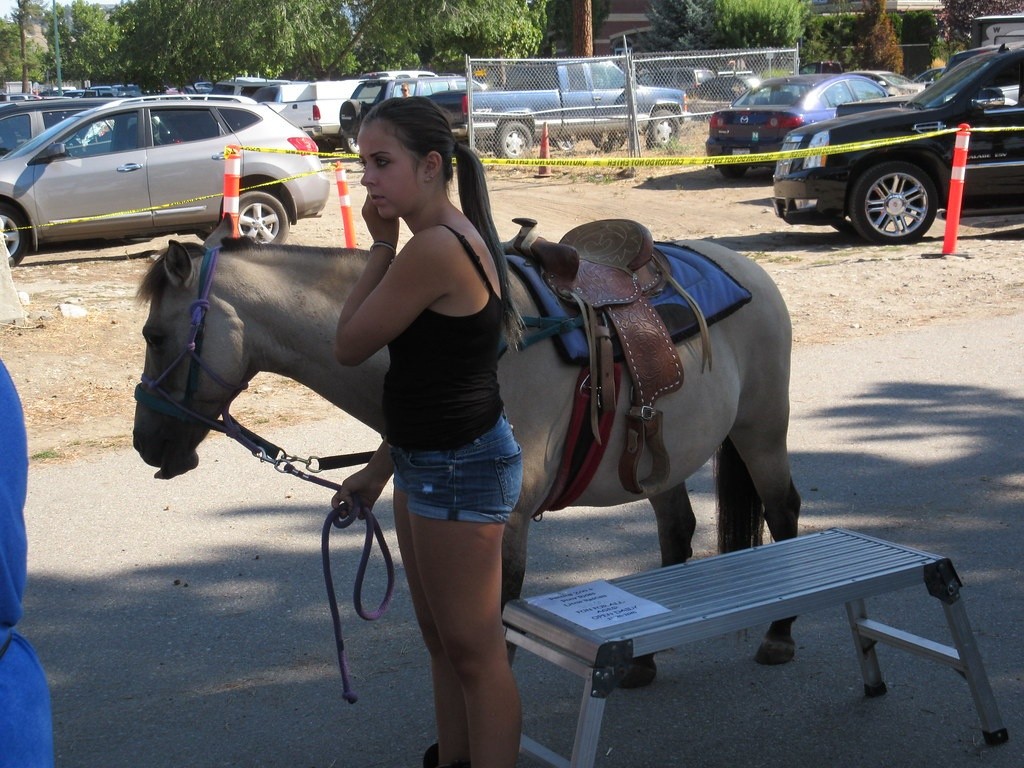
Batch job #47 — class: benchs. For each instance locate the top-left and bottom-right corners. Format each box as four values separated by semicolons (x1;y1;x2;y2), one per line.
497;525;1008;768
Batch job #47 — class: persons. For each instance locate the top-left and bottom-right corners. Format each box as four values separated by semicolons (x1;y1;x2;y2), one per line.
329;93;524;768
1;358;57;768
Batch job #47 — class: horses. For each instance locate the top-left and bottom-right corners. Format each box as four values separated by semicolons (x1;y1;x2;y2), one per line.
132;214;802;689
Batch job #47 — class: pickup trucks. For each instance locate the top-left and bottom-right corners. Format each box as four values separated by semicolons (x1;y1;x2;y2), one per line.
260;95;364;155
431;57;695;159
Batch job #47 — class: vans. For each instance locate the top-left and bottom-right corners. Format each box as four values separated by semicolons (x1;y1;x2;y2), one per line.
637;66;717;92
359;69;438;80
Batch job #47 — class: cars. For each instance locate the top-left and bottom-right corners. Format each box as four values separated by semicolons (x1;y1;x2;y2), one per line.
685;75;771;103
841;69;927;97
705;71;895;180
0;76;297;116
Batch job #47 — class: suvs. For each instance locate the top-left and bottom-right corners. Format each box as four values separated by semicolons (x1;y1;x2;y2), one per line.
340;74;489;146
0;89;331;267
0;95;172;161
770;41;1024;245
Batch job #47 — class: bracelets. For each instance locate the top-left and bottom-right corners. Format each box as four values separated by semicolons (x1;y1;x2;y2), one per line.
370;239;397;264
